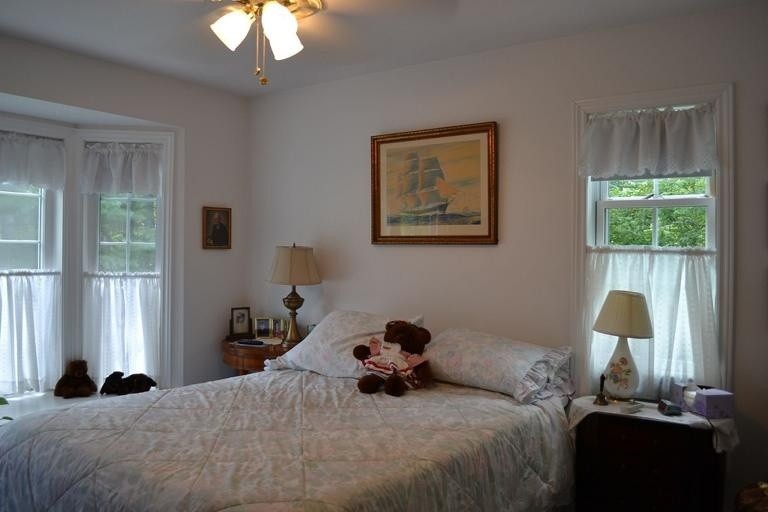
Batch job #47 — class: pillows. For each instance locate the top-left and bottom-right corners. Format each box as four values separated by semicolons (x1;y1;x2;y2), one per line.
423;327;573;404
264;309;422;378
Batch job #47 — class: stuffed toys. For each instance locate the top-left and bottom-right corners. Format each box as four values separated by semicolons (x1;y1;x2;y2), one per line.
55;360;97;399
353;320;433;397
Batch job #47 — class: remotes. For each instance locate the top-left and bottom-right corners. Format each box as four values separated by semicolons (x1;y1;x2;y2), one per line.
238;341;264;346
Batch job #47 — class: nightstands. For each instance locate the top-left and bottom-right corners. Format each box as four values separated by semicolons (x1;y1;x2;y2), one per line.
567;395;726;512
221;337;299;377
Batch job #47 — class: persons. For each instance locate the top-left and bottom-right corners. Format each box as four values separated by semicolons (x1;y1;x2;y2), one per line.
211;212;228;247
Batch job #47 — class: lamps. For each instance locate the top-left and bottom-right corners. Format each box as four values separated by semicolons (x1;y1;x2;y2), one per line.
591;289;653;402
209;1;306;87
265;242;321;348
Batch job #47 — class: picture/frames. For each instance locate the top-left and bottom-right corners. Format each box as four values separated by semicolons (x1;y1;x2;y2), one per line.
255;316;270;338
271;318;285;339
231;307;251;336
370;120;498;246
201;206;232;250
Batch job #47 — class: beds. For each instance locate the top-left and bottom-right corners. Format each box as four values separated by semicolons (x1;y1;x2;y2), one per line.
0;363;596;512
